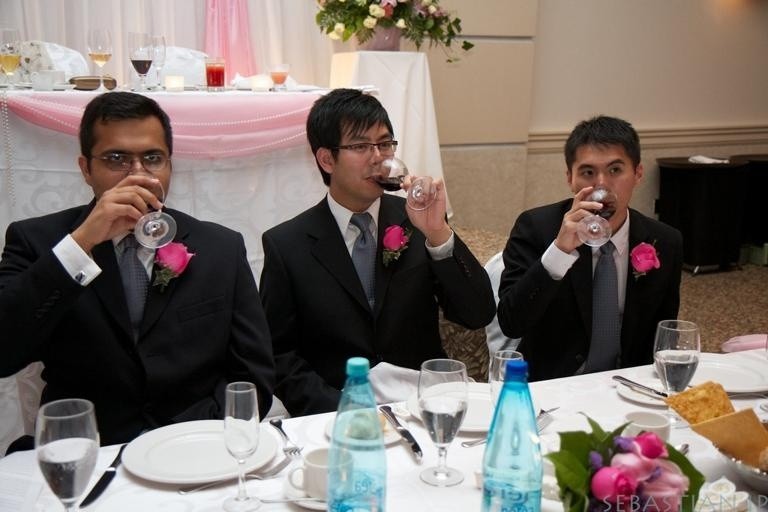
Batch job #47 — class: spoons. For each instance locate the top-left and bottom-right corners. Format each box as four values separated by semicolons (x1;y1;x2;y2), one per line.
269;418;290;441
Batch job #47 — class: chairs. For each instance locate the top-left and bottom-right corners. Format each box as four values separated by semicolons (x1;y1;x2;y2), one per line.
131;45;209;87
481;244;520;378
7;39;90;87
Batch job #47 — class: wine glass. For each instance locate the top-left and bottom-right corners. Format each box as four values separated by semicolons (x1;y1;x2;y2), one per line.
222;382;263;512
34;398;100;512
576;186;618;247
147;36;166;91
127;32;153;92
0;28;24;91
126;169;177;249
86;26;113;79
417;358;470;488
653;319;701;428
372;156;437;211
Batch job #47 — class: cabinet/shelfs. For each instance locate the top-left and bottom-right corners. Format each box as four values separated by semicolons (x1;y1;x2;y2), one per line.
730;154;768;267
655;154;742;276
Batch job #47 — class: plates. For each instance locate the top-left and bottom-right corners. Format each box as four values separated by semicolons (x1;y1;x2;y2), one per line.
286;477;329;512
325;407;408;447
120;419;278;485
406;381;542;432
616;378;691;407
24;82;76;90
688;353;768;393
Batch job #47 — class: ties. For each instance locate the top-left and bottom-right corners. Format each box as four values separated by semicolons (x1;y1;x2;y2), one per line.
583;240;620;374
350;212;377;311
119;234;149;345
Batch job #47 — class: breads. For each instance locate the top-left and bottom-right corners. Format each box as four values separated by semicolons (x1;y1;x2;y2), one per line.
667;382;733;416
689;405;768;470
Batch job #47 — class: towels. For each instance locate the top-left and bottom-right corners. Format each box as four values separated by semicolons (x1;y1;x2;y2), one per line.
334;360;475;409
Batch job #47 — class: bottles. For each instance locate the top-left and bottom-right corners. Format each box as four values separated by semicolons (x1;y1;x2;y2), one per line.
480;360;544;511
325;357;387;512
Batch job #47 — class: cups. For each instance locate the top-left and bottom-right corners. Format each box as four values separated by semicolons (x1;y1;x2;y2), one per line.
288;447;355;502
488;350;524;409
31;69;65;86
270;64;291;92
624;410;670;443
205;56;225;92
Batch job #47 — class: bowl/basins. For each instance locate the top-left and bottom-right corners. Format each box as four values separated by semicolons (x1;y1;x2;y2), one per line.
716;422;768;495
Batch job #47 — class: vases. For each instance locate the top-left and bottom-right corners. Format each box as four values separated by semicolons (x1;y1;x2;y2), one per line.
365;22;404;53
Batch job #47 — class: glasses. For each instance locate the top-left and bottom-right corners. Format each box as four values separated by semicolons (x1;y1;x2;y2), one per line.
91;153;171;171
338;141;398;155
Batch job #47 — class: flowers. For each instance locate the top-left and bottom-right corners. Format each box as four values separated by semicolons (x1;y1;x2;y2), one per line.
628;241;660;280
314;0;476;62
544;409;702;512
380;222;412;268
150;241;193;290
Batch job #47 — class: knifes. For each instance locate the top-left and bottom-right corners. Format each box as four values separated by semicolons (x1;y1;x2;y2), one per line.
79;443;127;508
379;405;423;458
612;375;668;400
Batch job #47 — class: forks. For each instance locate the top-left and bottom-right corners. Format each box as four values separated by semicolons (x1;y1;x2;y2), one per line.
177;446;304;495
461;412;554;448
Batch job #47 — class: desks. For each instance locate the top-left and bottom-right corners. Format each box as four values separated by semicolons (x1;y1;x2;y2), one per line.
332;53;433;214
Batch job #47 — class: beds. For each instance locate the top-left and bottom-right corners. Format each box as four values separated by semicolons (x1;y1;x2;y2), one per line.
1;343;768;512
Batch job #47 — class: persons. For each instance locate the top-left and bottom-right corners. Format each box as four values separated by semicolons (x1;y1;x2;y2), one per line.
498;116;682;382
258;87;496;417
0;91;275;455
